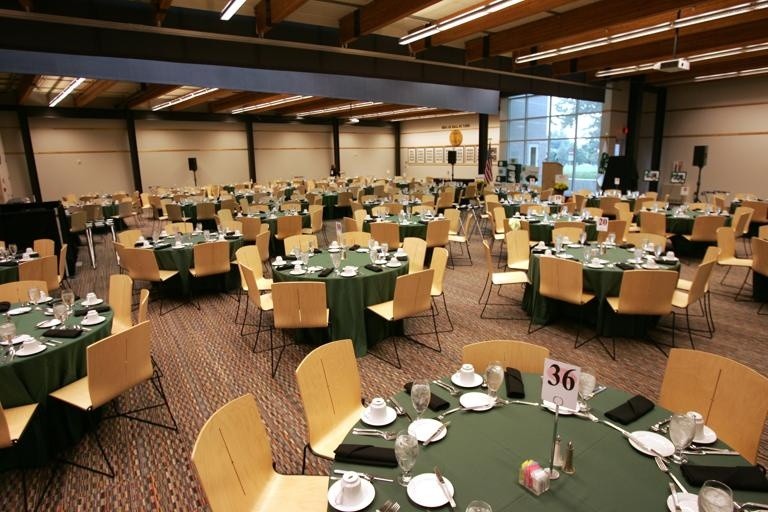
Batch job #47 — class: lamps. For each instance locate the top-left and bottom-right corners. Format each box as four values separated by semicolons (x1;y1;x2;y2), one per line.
46;77;479;125
216;0;766;82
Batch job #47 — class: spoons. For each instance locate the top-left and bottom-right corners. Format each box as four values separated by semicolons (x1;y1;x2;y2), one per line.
434;378;463;397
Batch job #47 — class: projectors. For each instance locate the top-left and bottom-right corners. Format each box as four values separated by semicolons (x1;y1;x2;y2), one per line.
653;58;691;73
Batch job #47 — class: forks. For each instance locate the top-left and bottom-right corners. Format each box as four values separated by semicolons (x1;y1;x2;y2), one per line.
387;396;407;417
655;457;689;496
351;428;400;441
375;499;401;512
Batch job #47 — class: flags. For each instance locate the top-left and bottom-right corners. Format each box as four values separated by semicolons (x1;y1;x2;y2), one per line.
484;144;493;183
597;138;609;186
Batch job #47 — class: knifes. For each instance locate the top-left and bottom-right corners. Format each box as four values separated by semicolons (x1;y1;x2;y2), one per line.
434;466;458;508
422;421;451;447
681;444;741;456
331;468;394;485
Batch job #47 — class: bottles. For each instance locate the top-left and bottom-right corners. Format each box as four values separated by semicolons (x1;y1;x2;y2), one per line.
548;431;576;475
485;359;505;397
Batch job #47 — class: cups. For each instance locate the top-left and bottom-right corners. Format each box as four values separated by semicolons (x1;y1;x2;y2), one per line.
689;411;704;439
460;362;476;383
26;248;33;254
369;398;387;420
23;253;30;260
335;471;363;507
39;291;46;301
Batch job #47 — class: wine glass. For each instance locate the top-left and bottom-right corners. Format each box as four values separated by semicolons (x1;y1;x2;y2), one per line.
29;288;43;311
696;480;734;512
394;434;417;487
61;289;76;315
579;232;587;248
412;377;431;417
669;409;693;464
608;232;616;247
9;244;18;260
577;367;596;412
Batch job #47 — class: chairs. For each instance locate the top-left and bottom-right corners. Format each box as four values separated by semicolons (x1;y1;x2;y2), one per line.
48;315;183;483
0;166;766;381
189;388;333;511
456;339;551;382
655;348;768;468
291;337;372;476
0;403;41;512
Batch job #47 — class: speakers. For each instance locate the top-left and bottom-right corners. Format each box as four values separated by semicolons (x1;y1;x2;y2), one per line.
189;158;197;170
448;151;456;163
693;145;708;166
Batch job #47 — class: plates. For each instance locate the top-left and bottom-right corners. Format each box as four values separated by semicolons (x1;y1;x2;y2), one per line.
407;472;455;508
408;419;447;442
0;298;107;358
360;407;397;427
327;479;376;512
458;391;493;413
24;252;37;255
534;240;680;270
273;240;409;277
29;297;53;304
18;258;35;262
691;425;717;444
665;492;750;512
626;430;676;457
134;223;243;250
450;372;483;389
